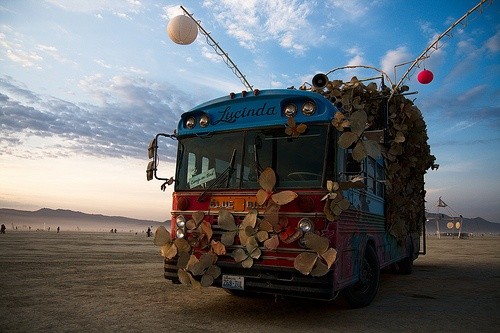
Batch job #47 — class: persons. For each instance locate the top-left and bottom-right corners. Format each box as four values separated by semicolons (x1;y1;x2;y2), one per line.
57;226;60;232
1;224;6;234
111;229;117;233
35;226;50;232
130;226;152;237
14;225;31;230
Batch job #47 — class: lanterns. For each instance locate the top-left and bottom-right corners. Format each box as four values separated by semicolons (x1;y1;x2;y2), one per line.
166;15;198;45
417;70;434;84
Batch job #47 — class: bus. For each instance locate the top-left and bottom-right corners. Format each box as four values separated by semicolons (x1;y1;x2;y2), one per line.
145;64;431;312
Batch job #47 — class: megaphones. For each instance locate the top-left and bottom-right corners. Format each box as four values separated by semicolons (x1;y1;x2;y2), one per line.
312;74;329;89
